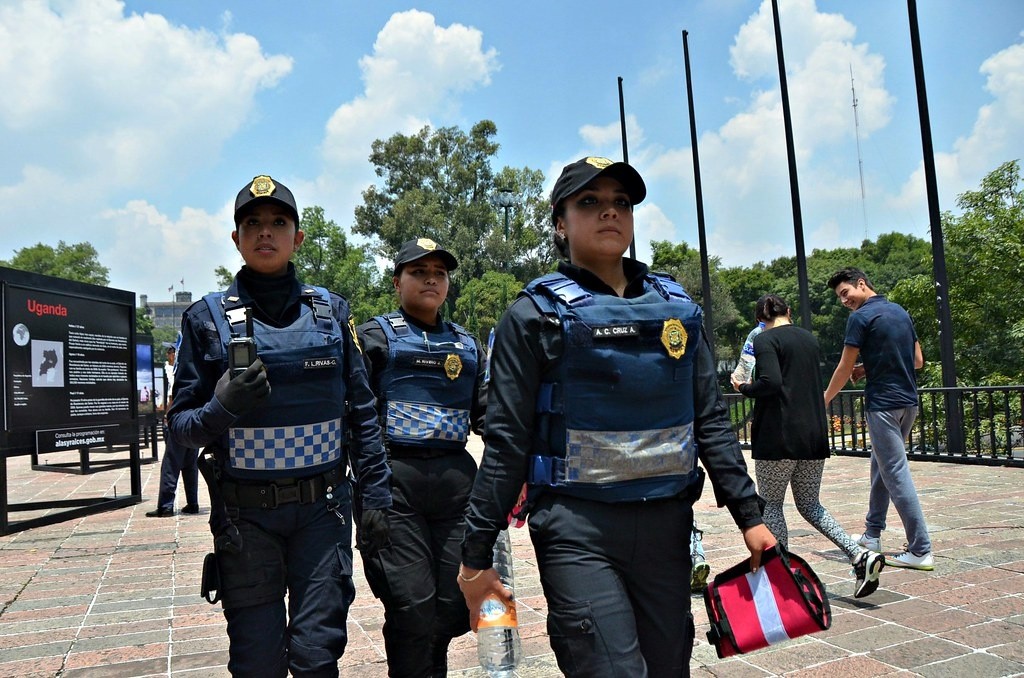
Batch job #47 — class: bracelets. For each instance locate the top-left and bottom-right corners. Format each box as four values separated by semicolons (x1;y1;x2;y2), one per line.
460;562;483;582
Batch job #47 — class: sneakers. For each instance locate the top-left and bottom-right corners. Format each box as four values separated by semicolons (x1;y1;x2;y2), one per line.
851;534;881;553
882;543;934;571
690;554;710;590
850;550;885;598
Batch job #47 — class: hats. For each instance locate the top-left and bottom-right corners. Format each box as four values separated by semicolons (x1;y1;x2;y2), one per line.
233;176;299;231
550;157;646;218
162;342;177;349
393;239;459;275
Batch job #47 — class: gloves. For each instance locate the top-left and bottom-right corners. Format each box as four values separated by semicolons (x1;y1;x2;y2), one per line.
214;358;272;417
356;510;391;556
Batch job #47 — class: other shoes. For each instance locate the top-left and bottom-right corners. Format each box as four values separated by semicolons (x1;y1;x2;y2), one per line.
145;509;173;517
182;504;199;513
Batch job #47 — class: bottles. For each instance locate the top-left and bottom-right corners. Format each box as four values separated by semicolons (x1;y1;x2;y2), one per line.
730;322;766;392
477;528;520;678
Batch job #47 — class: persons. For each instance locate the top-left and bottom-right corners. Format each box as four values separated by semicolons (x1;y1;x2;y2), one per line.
146;348;199;517
141;387;149;403
351;239;525;678
824;267;933;569
730;295;885;598
690;531;710;593
457;157;781;678
164;173;391;678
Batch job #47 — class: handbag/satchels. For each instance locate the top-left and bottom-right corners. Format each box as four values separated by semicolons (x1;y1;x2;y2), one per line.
703;544;832;659
